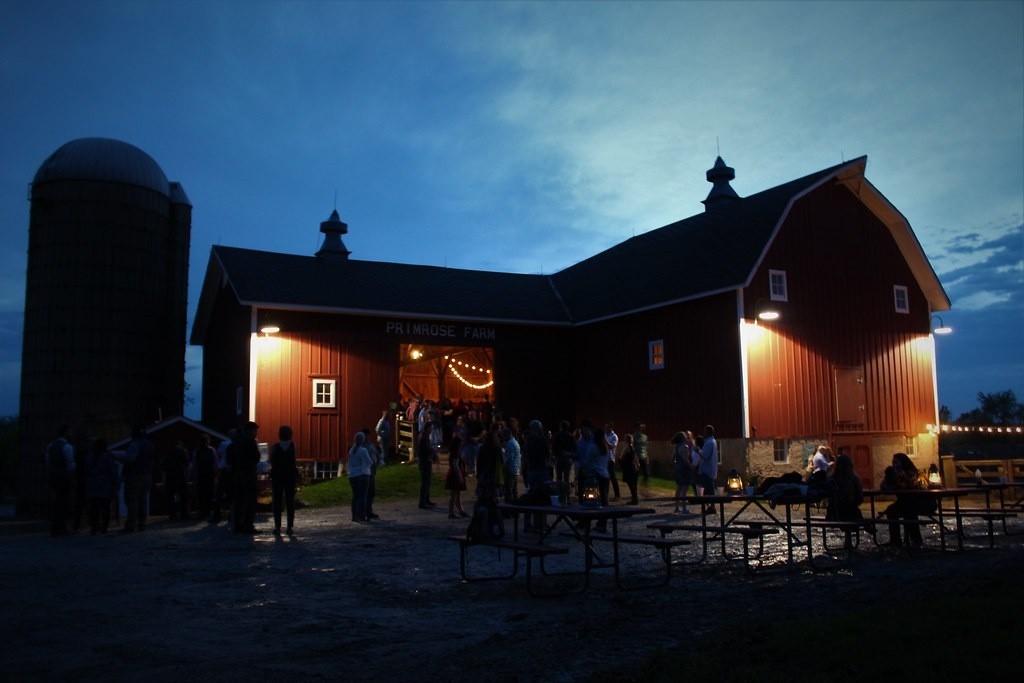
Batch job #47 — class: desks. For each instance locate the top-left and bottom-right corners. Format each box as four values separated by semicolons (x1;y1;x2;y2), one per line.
758;487;839;564
640;494;768;579
860;483;1024;551
495;501;655;590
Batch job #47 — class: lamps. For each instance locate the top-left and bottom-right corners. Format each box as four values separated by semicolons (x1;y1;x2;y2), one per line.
580;479;603;510
928;464;944;490
727;468;744;497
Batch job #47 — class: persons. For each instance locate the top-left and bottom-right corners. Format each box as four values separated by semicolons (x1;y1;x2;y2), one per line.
39;393;983;553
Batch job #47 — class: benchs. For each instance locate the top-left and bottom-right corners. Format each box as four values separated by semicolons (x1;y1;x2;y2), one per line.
646;520;779;580
558;529;690;588
449;535;580;600
804;500;1024;547
719;528;780;568
735;520;871;564
